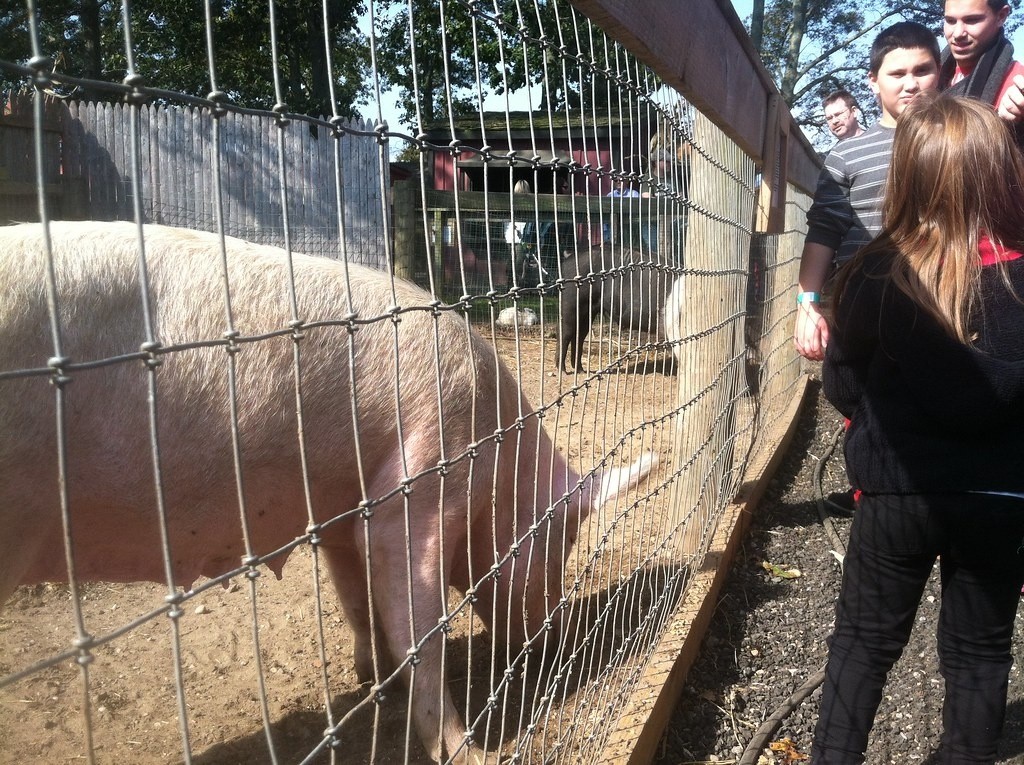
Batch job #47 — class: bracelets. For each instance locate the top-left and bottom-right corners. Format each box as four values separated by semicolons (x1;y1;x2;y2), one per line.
796;291;821;304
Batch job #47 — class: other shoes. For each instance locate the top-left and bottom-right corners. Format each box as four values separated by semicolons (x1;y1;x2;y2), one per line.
827;490;857;515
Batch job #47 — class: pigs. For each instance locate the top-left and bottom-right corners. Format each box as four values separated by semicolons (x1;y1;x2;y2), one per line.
554;245;765;434
2;218;660;765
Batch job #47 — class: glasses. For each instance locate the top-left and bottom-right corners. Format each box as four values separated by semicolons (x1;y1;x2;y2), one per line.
562;186;568;189
612;179;621;182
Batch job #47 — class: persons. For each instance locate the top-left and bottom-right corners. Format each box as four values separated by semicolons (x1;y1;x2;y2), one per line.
504;148;682;298
794;0;1024;764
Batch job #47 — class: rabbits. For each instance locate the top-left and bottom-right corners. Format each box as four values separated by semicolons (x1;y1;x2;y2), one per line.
494;305;539;330
